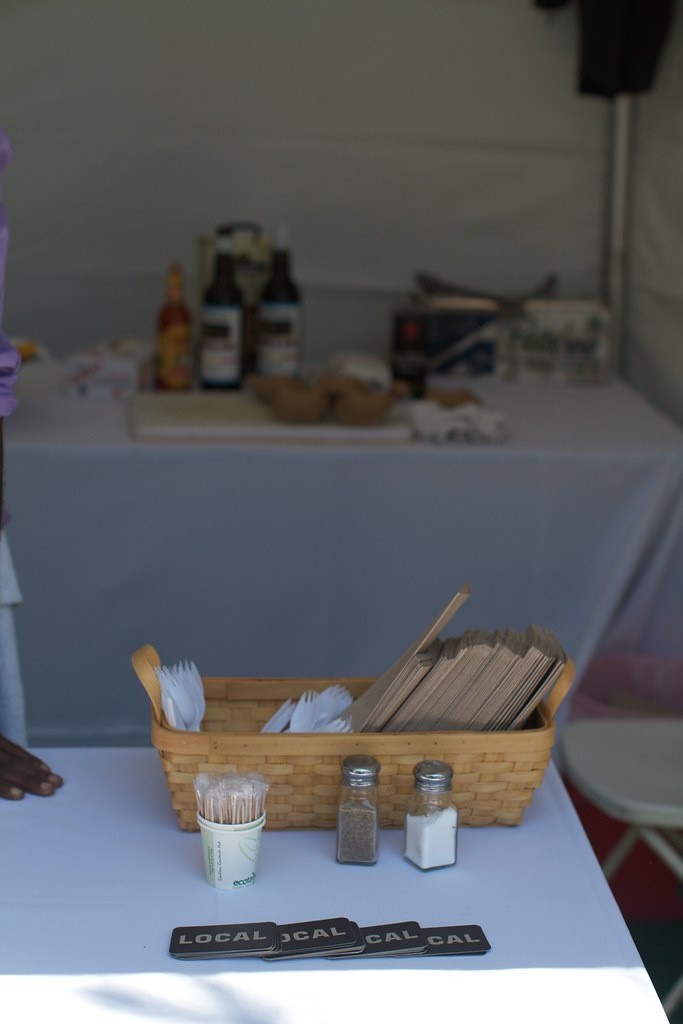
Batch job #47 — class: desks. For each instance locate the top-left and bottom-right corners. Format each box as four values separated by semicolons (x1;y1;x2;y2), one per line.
0;748;670;1024
0;354;682;779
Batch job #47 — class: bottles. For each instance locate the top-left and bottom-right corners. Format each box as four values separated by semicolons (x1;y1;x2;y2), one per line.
252;246;302;383
402;759;458;870
389;324;426;399
335;754;378;866
157;262;194;391
198;238;247;391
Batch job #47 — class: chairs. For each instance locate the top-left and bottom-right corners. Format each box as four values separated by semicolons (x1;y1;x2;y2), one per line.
559;720;683;1021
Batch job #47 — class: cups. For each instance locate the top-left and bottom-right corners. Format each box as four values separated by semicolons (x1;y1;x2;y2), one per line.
196;811;267;890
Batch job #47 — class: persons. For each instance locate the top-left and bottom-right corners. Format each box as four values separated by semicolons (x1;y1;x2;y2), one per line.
0;134;65;798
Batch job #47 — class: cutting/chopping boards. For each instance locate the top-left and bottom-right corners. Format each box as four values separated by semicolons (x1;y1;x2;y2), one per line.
130;393;415;447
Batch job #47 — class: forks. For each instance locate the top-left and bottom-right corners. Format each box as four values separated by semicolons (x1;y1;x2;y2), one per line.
153;660;206;735
258;685;356;734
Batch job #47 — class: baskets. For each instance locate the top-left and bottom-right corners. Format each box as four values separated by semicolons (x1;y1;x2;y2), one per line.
131;645;576;832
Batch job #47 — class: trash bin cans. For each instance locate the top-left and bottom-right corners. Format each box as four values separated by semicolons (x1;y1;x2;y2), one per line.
565;654;683;921
